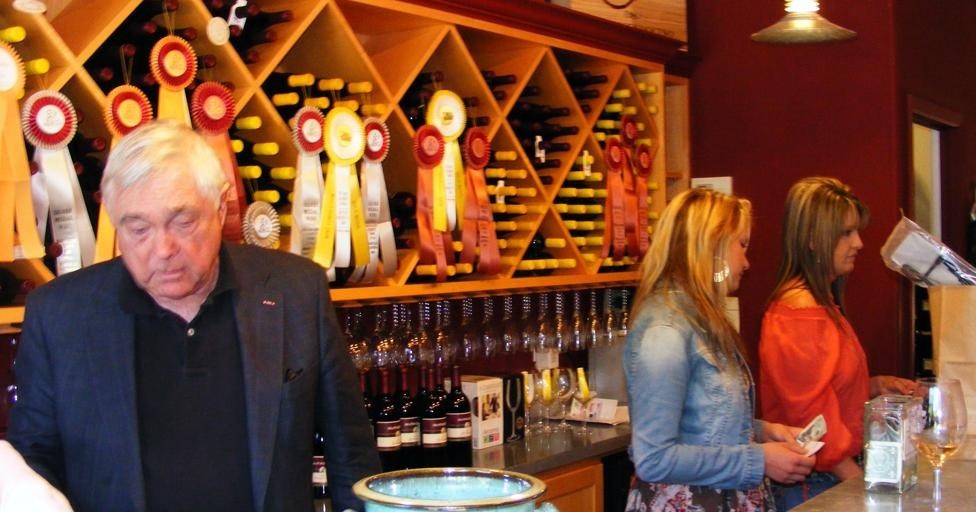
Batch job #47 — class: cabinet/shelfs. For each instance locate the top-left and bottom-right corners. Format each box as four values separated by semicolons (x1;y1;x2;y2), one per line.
532;461;607;512
0;1;696;319
906;280;936;383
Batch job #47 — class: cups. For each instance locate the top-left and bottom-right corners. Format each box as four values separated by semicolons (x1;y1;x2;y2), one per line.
514;432;593;468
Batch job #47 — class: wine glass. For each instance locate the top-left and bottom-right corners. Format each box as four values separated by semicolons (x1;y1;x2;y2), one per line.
506;378;523;443
344;286;641;373
906;377;968;512
519;367;597;435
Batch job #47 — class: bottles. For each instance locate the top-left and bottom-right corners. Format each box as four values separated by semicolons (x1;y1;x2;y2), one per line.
0;334;20;441
0;0;660;306
311;360;474;500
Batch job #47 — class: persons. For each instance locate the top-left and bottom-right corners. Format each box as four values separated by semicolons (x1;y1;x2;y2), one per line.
754;175;931;512
7;120;384;512
621;187;817;512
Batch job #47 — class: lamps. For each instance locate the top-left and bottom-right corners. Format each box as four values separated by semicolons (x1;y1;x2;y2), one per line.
750;0;858;46
